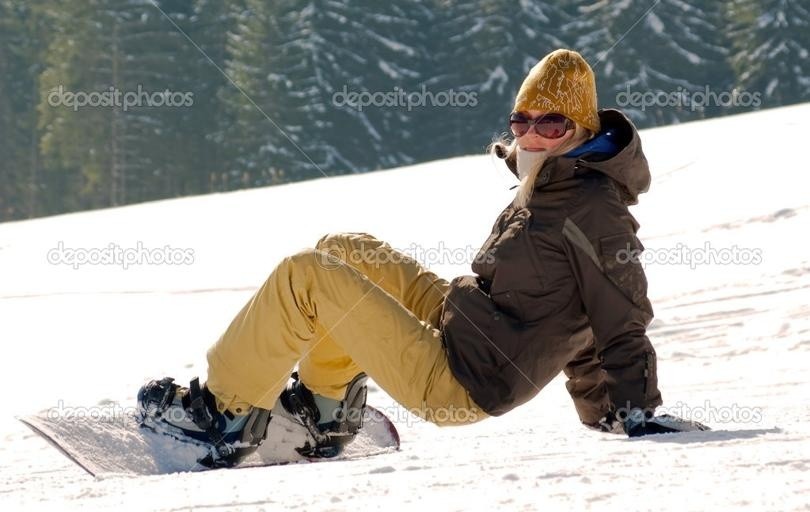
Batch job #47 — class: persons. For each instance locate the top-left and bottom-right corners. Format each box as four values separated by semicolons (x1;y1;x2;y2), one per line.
138;47;709;454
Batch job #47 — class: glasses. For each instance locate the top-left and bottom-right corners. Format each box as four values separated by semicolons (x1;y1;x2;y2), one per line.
509;112;574;139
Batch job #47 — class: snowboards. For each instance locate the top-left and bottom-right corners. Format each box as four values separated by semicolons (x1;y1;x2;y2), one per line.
18;402;399;478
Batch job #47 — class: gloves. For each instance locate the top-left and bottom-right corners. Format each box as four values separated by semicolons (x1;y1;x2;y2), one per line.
594;410;710;437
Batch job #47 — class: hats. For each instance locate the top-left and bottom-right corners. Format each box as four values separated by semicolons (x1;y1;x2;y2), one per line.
514;49;601;132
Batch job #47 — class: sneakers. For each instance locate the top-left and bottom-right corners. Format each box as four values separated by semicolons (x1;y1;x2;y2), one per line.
272;377;343;430
138;380;245;441
